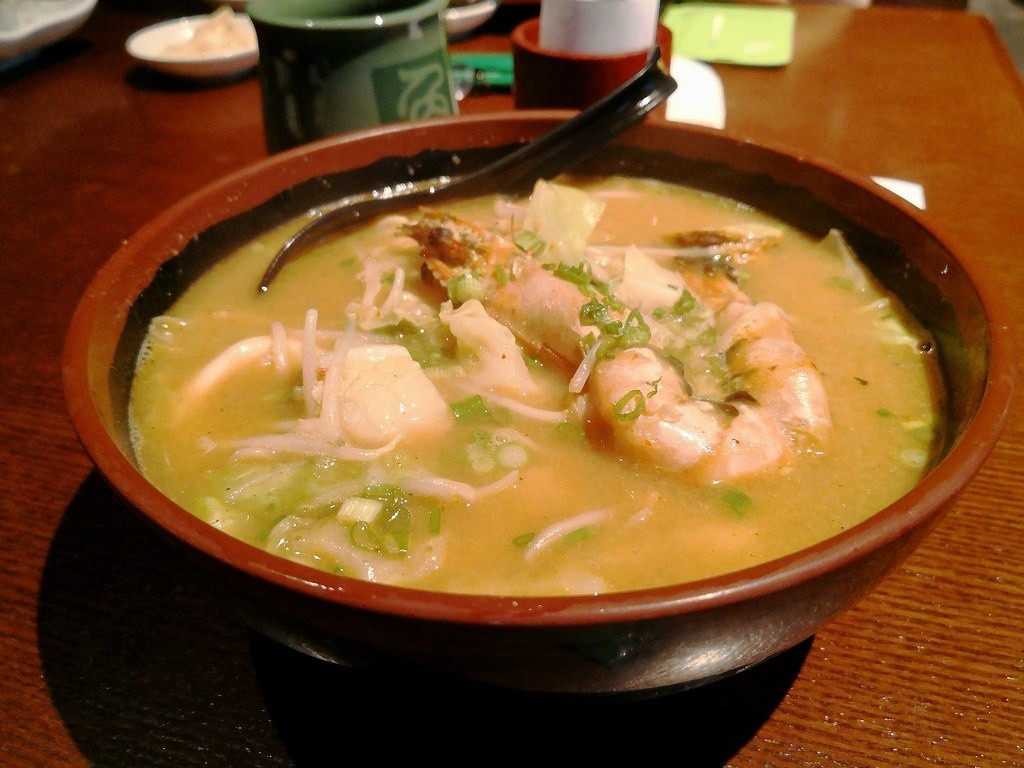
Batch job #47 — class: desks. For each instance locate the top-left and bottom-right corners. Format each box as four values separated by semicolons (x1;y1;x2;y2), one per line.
0;1;1022;767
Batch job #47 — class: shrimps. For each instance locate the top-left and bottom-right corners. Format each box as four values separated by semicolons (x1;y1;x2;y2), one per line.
396;205;659;366
598;275;829;486
682;224;780;259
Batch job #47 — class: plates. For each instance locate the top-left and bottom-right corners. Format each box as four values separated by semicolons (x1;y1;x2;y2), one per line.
124;13;259;80
444;0;500;42
0;0;97;69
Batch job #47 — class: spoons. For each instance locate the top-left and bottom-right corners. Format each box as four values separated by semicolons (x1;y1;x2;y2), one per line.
257;48;679;298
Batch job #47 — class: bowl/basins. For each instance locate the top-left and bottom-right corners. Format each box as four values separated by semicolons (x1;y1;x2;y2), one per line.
59;107;1014;699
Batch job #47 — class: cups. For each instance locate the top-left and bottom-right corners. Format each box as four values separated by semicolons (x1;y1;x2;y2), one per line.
510;15;672;118
244;0;460;156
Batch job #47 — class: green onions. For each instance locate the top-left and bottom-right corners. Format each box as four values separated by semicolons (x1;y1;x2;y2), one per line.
187;206;943;563
